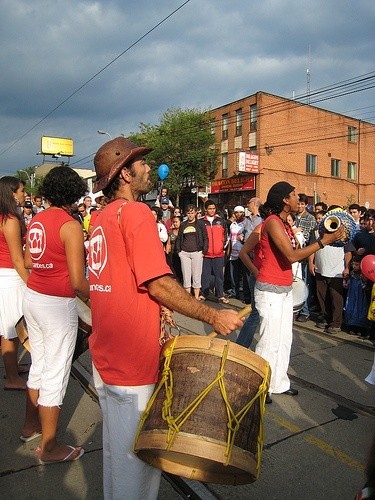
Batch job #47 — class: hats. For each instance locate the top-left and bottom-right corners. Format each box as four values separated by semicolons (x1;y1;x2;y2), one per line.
234;206;244;212
92;136;153;194
267;181;295;203
25;201;32;207
161;198;169;204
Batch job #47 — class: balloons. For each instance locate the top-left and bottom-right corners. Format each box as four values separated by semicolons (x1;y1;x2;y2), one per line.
158;164;169;180
360;255;375;282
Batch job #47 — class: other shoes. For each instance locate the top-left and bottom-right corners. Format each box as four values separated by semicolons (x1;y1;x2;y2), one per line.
198;295;207;301
223;289;237;298
265;392;272;404
217;297;230;304
318;319;341;334
282;389;298;396
297;314;310;322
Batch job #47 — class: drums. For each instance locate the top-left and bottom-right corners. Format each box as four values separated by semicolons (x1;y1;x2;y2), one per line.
136;336;272;485
15;293;91;364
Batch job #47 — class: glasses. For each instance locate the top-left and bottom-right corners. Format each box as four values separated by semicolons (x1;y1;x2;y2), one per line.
79;209;84;213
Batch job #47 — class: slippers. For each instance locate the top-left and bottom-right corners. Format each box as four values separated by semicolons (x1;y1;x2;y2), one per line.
4;369;27;390
20;431;42;442
39;445;84;464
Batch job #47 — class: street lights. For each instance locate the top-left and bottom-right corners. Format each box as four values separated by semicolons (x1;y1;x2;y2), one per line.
19;169;33;203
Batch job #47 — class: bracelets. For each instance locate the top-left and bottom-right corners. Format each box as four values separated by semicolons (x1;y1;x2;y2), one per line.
318;240;324;249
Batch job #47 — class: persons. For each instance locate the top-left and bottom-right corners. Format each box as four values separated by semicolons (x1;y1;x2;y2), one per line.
0;176;31;391
33;196;45;214
236;203;270;346
155;186;174;208
69;196;104;280
22;201;35;223
152;198;264;305
287;193;375;341
89;136;246;500
254;181;345;404
22;166;90;464
